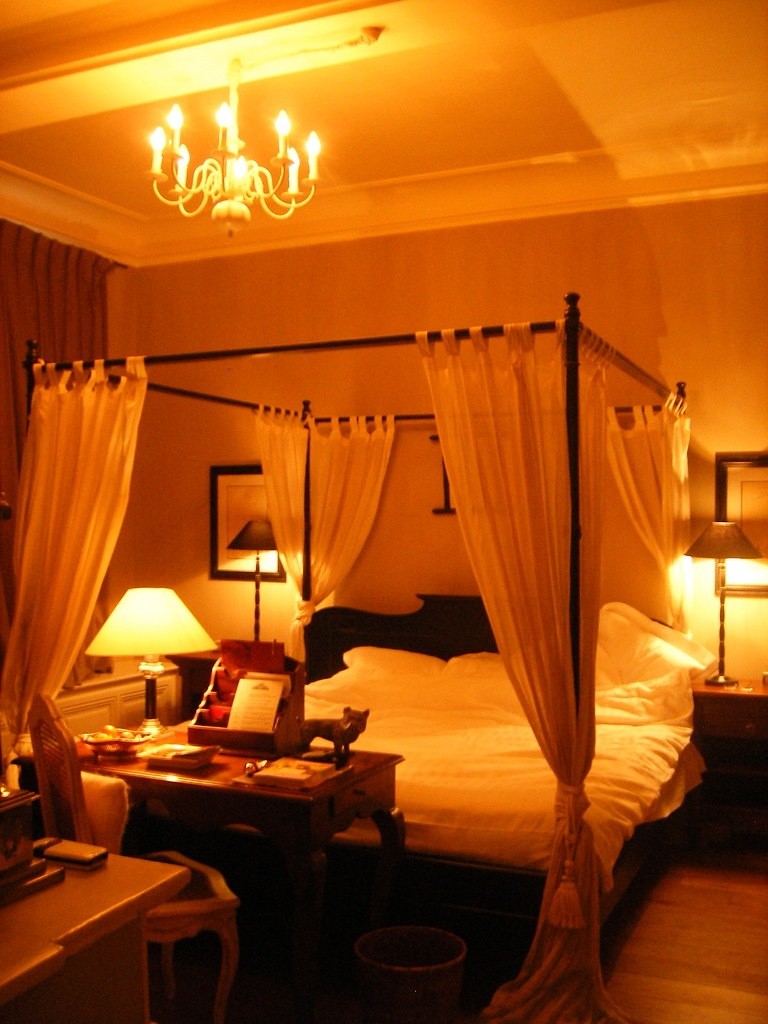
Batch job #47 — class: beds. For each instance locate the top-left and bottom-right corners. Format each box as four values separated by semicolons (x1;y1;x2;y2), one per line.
120;594;673;981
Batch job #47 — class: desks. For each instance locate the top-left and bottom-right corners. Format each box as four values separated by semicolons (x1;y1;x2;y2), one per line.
0;840;191;1024
10;725;406;1024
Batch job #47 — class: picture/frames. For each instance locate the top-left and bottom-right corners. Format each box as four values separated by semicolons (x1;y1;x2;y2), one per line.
715;451;768;599
208;464;287;583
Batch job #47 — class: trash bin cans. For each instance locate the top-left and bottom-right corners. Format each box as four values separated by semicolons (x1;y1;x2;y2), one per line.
357;927;466;1024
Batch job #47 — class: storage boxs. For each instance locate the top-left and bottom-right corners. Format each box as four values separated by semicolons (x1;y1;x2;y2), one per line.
188;656;306;759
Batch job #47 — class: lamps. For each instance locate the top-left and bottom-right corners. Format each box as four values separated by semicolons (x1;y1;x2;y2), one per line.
225;520;277;640
84;587;220;740
147;60;322;238
683;522;762;686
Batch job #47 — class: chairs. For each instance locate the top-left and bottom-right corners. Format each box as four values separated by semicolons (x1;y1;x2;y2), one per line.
27;693;241;1023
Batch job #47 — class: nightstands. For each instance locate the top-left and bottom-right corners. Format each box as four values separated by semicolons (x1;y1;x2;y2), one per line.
688;678;768;870
166;649;223;719
